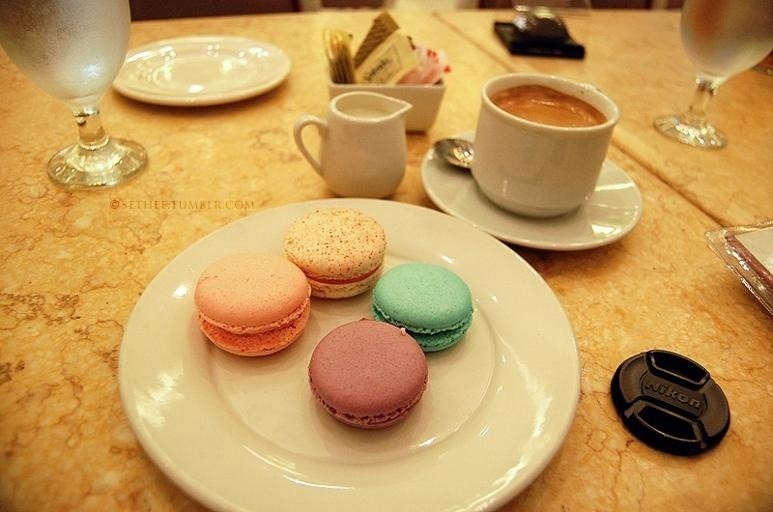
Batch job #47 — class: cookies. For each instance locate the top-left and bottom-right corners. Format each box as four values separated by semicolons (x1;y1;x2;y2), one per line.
308;318;429;430
371;262;474;353
194;252;311;358
283;207;387;300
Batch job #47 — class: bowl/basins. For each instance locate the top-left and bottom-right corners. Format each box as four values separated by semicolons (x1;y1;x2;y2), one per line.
324;74;446;135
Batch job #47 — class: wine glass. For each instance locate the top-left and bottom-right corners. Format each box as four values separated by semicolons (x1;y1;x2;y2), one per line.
0;0;152;189
650;3;771;151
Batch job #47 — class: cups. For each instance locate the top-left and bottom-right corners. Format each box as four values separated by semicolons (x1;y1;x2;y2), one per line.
294;91;412;195
473;78;619;210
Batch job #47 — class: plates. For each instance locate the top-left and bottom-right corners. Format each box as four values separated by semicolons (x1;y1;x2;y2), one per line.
107;37;291;111
421;135;645;252
118;196;583;509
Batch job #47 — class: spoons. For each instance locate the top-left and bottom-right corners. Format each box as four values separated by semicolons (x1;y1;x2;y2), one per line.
435;132;478;177
